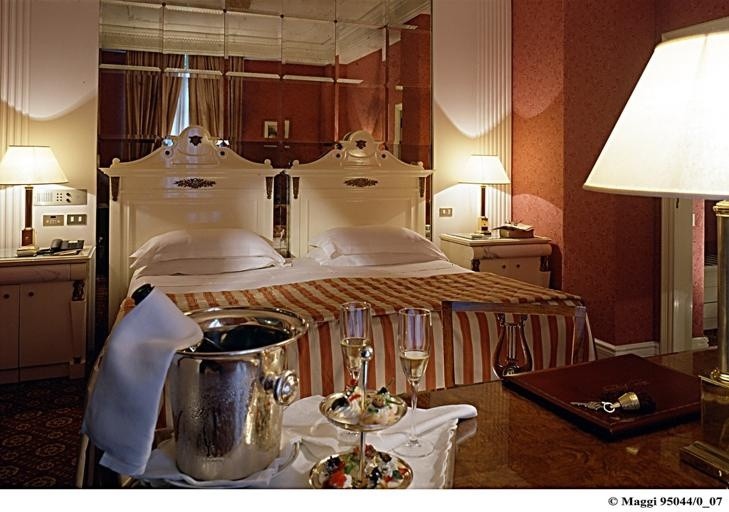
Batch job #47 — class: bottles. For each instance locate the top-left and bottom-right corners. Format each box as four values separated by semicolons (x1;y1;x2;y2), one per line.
131;284;229;352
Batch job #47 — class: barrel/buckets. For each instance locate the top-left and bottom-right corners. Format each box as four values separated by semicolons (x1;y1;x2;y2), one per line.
169;305;310;482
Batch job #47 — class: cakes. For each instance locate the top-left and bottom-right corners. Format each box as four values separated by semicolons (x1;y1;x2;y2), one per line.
324;385;398;423
319;443;407;489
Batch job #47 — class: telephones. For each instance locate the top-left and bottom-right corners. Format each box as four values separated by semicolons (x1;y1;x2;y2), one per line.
51;239;84;251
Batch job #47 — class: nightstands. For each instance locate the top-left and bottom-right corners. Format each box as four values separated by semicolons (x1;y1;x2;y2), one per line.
438;231;553;289
0;245;96;384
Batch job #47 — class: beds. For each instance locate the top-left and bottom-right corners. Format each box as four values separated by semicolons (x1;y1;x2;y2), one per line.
97;123;590;400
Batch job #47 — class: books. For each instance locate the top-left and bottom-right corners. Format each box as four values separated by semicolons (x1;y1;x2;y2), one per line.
445;230;488;240
491;222;534;232
499;228;536;239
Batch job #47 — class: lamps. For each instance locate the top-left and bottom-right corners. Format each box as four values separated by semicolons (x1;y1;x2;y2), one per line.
0;145;69;257
457;153;512;237
584;28;729;485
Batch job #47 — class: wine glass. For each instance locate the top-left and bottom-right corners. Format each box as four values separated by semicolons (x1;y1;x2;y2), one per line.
392;307;436;457
335;301;375;444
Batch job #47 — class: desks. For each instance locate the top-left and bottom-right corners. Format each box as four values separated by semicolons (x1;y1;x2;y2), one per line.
146;347;728;489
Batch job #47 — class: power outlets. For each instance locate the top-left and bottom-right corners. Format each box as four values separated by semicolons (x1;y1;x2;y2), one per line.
43;215;65;227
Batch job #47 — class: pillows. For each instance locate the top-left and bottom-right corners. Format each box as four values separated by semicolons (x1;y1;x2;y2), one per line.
307;225;449;260
305;247;435;267
128;227;286;269
132;257;282;280
